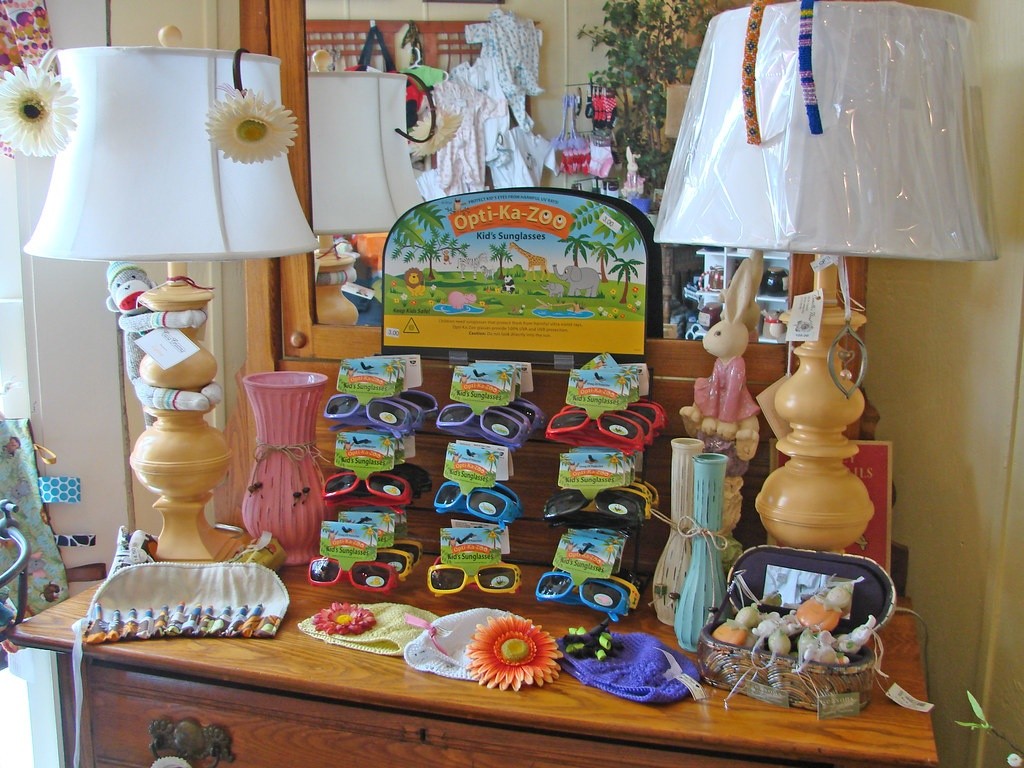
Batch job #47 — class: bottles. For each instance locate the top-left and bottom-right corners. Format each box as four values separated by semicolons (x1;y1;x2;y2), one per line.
243;371;333;569
652;437;729;653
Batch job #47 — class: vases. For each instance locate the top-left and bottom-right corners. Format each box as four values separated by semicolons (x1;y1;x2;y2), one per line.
652;438;703;627
243;372;324;565
674;452;728;652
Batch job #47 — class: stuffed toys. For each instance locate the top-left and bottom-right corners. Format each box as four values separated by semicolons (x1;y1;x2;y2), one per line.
106;261;223;429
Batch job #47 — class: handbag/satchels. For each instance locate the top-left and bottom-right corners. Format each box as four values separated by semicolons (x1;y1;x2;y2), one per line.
83;563;290;644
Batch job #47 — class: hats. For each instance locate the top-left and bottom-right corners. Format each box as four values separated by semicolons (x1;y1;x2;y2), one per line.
556;626;707;703
298;602;442;657
402;608;564;692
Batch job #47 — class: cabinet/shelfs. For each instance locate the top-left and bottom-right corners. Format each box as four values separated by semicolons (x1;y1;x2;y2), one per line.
10;553;938;768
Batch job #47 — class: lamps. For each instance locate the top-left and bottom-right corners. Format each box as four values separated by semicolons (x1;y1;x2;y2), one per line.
308;49;422;325
24;25;319;563
654;0;999;552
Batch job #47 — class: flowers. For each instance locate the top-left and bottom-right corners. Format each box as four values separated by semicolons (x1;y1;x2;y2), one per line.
205;47;298;163
465;612;563;693
0;47;78;158
311;602;376;634
409;109;464;156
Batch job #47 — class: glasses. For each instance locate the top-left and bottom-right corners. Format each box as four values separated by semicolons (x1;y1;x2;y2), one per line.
307;390;669;620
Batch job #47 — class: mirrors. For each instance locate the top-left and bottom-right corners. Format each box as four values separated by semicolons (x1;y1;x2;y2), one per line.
269;0;793;382
